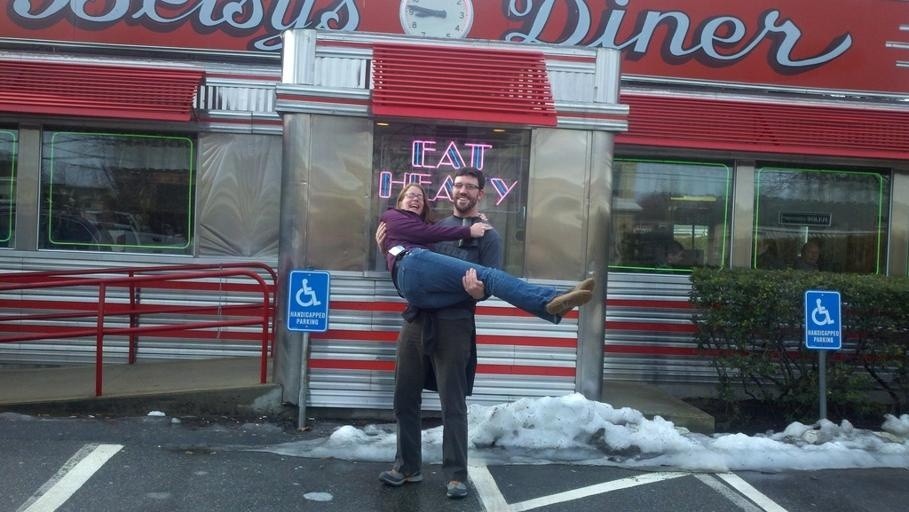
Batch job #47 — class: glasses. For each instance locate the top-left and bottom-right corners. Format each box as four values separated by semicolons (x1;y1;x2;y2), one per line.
403;192;426;200
453;182;480;191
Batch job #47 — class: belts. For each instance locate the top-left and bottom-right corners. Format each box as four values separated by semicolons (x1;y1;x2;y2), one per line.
391;249;406;298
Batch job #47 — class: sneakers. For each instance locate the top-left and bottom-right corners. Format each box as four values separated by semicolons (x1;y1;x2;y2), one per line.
378;463;423;486
445;479;467;498
545;278;595;317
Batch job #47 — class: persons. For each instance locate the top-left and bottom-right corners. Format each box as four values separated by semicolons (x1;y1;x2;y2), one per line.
375;167;501;498
379;183;596;324
793;242;820;272
650;241;684;274
